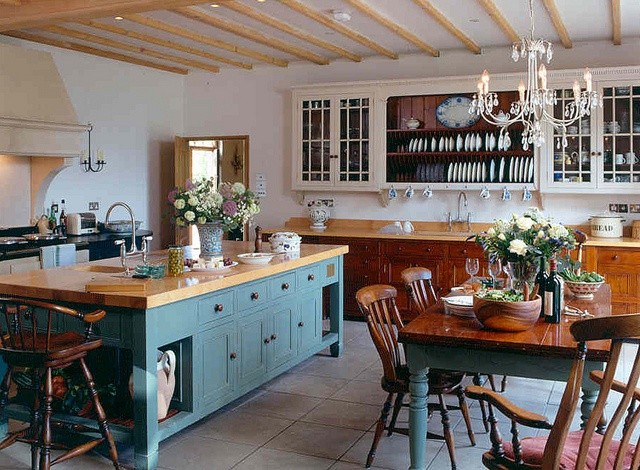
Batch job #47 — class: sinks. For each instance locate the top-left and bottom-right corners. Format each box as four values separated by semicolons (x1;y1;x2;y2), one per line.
62;265;131;273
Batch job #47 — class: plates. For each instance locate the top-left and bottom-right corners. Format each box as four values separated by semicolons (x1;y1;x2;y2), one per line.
475;133;482;152
482;161;487;182
472;161;476;182
528;156;534;183
465;133;469;152
418;138;423;152
458;162;462;182
439;137;444;152
436;97;481;128
449;137;455;152
191;261;238;272
409;139;413;152
477;162;481;182
514;156;519;182
523;156;529;182
424;138;428;152
413;138;418;152
519;157;524;182
431;136;436;152
453;162;458;182
485;133;489;151
445;137;449;152
447;163;453;182
489;133;496;151
509;157;514;183
456;134;463;152
470;133;475;152
490;159;497;182
440;296;477;317
467;162;471;182
499;157;505;182
462;162;467;182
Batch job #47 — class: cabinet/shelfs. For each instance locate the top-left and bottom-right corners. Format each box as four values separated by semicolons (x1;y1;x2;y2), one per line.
267;267;323;382
196;279;266;414
450;242;505;287
540;66;640;195
558;246;597;272
381;72;539;202
301;236;379;317
377;238;450;324
291;79;382;192
597;245;640;339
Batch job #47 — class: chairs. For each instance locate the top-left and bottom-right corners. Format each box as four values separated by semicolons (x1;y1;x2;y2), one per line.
465;313;640;469
400;266;497;431
355;285;475;469
1;297;122;470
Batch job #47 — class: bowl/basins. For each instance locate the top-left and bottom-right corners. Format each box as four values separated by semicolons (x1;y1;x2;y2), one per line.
565;281;605;300
473;290;543;332
237;253;275;265
101;220;144;232
582;118;590;135
268;232;303;253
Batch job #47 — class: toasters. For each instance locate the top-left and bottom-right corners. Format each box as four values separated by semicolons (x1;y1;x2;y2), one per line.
67;212;99;236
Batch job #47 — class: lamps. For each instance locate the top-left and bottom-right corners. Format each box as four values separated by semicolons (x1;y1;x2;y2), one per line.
475;38;603;151
80;125;106;173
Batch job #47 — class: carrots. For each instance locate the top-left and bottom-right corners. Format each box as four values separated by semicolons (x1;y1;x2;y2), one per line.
524;281;530;300
529;283;540;300
504;278;508;287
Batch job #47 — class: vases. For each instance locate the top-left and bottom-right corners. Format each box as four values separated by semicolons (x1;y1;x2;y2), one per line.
193;219;225;259
506;261;533;291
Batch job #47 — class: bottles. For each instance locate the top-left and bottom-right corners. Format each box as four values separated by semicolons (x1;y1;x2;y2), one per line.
545;257;561;323
60;200;67;231
535;257;549;317
49;206;56;230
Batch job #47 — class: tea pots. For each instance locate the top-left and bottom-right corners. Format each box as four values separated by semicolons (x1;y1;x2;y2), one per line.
404;117;420;129
493;110;511;127
605;121;621;133
36;214;56;234
571;145;589;167
130;349;175;421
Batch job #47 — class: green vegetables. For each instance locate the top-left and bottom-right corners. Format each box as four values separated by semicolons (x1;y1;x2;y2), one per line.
560;270;604;281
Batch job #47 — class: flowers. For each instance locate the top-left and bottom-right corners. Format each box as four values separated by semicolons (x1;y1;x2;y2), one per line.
165;176;260;232
466;209;576;263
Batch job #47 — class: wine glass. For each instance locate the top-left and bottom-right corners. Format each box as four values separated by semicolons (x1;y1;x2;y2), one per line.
465;257;480;293
503;262;518;290
488;254;502;291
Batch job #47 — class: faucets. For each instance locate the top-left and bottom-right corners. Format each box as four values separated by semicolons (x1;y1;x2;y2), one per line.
105;203;137;256
454;192;468;222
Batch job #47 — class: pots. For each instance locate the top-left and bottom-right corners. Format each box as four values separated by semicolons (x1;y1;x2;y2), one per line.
588;213;626;238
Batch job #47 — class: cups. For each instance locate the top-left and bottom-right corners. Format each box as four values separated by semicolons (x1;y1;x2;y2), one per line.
615;154;625;164
625;153;639;164
403;221;414;233
